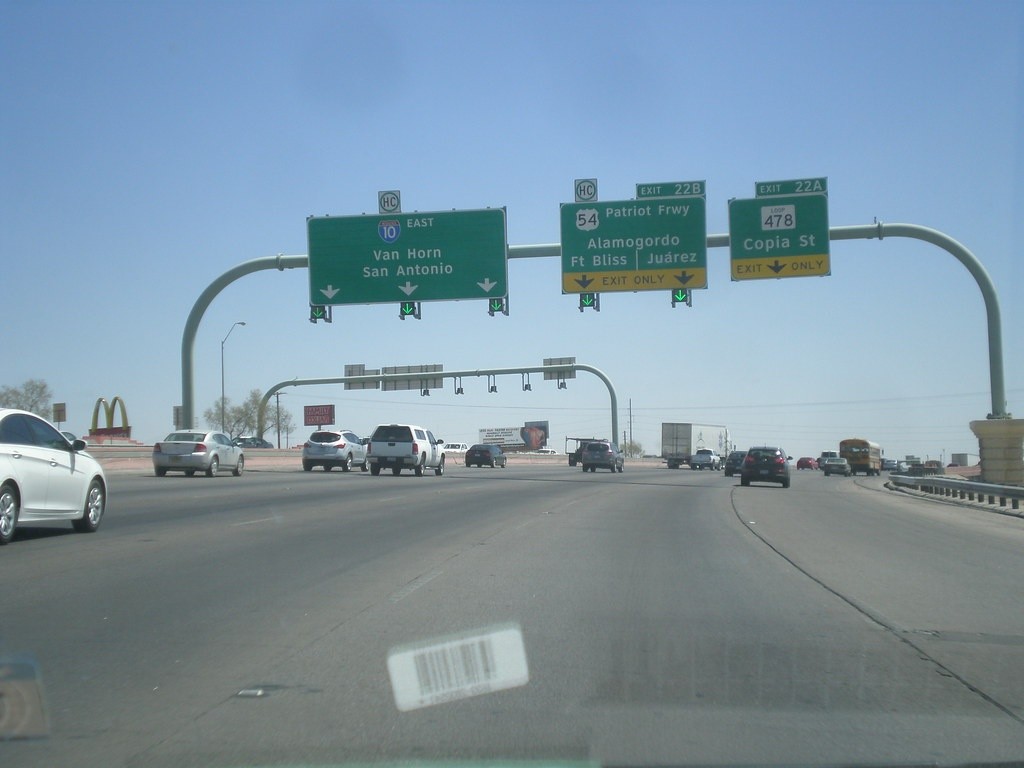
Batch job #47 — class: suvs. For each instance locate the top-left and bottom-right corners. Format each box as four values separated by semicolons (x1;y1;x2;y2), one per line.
581;442;624;474
302;428;369;473
740;446;794;488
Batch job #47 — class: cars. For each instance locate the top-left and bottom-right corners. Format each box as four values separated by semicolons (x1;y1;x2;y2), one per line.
152;430;244;478
0;408;108;547
232;436;274;448
445;442;558;469
797;457;959;478
60;430;88;450
725;450;748;477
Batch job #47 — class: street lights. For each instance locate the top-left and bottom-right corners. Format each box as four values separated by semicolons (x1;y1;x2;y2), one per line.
221;321;248;432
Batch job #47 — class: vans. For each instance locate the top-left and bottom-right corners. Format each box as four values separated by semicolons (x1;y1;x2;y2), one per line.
820;451;841;470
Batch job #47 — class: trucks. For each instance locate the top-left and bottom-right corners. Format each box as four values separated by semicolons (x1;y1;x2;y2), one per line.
565;437;608;467
660;422;737;470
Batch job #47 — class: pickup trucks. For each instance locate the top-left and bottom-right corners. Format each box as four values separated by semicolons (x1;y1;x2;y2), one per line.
366;423;446;476
691;448;723;472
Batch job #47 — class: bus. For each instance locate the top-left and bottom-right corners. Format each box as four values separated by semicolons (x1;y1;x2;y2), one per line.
839;439;885;477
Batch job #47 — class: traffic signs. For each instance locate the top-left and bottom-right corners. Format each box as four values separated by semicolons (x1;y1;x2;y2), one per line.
559;195;709;294
305;205;509;306
727;195;832;282
